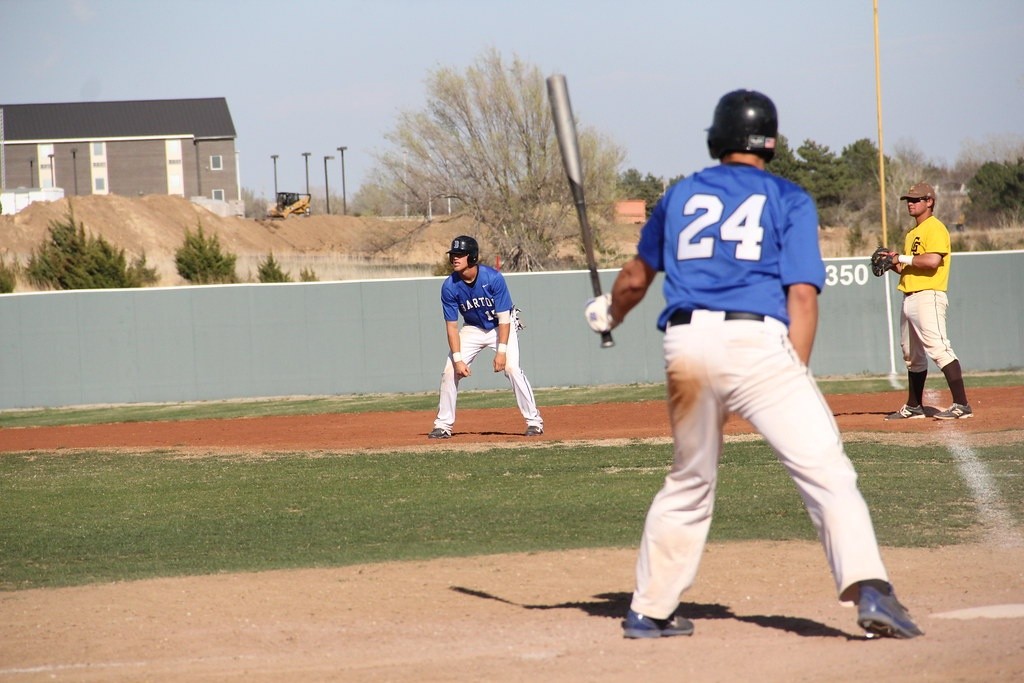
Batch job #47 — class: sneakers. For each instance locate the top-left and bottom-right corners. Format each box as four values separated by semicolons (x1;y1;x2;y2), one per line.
933;403;973;419
428;428;451;439
883;404;925;421
624;604;693;639
856;577;924;638
525;425;543;436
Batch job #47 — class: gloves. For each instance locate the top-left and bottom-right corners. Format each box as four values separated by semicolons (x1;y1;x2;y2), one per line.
586;291;619;334
511;303;526;332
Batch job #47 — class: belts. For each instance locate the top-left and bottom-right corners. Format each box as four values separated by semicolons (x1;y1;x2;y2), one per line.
670;310;764;326
904;290;923;297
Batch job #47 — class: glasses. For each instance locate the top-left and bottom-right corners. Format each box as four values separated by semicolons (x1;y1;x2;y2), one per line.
906;198;929;203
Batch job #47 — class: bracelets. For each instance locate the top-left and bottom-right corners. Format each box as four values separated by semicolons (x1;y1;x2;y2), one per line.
899;255;914;265
497;344;506;352
453;353;461;363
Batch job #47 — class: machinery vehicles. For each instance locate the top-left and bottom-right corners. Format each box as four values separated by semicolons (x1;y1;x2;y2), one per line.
267;192;312;219
943;212;965;232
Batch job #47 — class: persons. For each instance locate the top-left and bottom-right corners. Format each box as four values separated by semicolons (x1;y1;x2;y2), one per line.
871;182;973;420
427;235;543;438
585;89;922;640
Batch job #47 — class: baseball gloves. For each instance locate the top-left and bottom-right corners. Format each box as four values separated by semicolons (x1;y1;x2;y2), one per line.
872;247;899;277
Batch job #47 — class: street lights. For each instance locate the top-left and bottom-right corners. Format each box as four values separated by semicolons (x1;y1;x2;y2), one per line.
401;142;451;217
337;146;347;216
271;155;279;198
48;154;55;187
302;152;312;194
30;159;34;188
70;148;79;195
324;156;335;214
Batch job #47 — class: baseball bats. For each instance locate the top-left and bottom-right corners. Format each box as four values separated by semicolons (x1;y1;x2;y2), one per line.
546;74;615;348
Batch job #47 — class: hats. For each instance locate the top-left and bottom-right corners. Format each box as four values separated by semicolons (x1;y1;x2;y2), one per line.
901;183;935;201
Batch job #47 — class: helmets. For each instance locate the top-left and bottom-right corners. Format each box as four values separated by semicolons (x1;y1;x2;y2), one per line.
705;90;777;163
447;235;478;268
